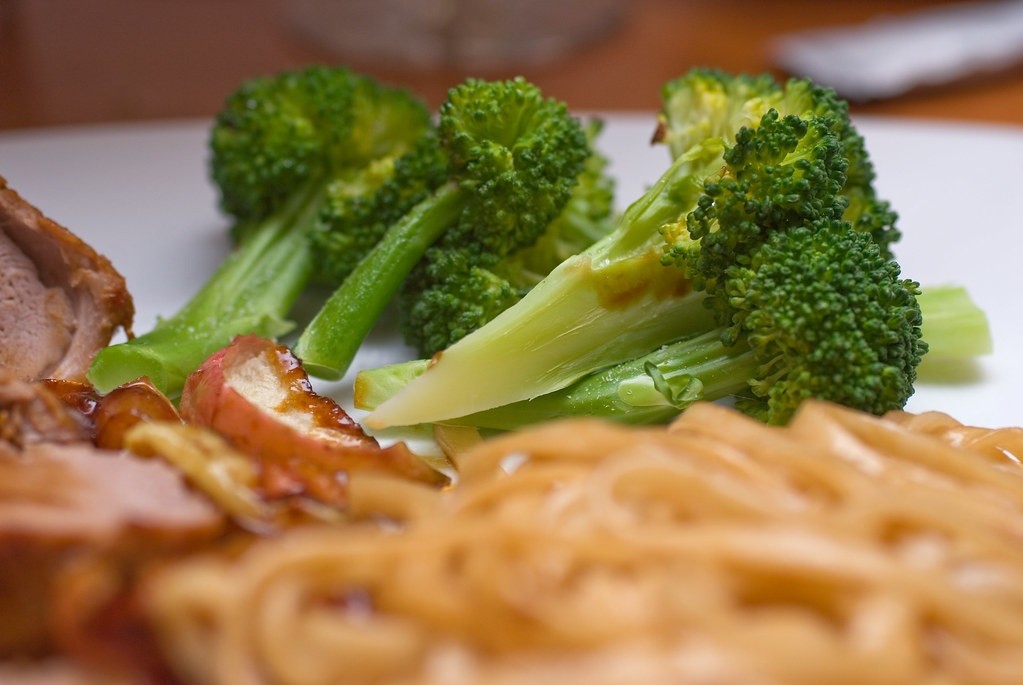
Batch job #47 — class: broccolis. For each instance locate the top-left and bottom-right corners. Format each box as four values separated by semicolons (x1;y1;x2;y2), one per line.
84;63;995;430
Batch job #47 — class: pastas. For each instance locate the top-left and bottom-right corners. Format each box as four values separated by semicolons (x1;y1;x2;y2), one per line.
124;396;1023;685
